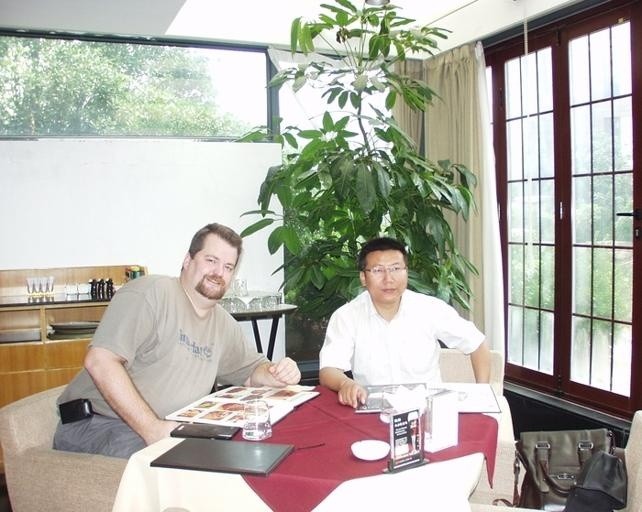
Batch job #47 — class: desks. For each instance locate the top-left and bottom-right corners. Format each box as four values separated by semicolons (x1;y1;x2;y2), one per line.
230;303;298;361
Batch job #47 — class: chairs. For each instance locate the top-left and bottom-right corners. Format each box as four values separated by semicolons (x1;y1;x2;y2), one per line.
0;385;132;512
439;348;505;383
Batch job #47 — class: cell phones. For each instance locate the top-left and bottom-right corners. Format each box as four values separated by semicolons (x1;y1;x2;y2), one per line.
169;423;240;440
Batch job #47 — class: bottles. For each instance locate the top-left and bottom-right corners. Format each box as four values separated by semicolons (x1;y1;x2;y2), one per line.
126;266;140;283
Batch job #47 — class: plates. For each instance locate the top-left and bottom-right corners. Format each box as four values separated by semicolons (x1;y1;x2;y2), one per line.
49;321;100;330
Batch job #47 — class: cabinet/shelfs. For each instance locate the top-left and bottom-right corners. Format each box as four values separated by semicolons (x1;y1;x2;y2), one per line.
0;265;149;407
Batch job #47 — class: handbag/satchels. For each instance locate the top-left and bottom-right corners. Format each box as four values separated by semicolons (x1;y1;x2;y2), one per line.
564;452;627;512
491;428;616;512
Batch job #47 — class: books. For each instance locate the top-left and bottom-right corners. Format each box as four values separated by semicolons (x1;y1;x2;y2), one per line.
151;437;299;479
353;382;503;417
164;383;320;430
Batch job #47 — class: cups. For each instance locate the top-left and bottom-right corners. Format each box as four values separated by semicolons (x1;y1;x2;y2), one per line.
220;279;282;313
378;386;407;423
242;398;273;441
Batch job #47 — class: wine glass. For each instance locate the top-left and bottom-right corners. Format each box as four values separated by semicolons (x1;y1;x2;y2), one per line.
26;277;55;296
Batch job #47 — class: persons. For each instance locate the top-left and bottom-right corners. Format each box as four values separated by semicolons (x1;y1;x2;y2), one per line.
317;237;494;408
49;221;303;462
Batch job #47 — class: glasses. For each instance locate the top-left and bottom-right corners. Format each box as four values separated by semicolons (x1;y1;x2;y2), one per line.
364;265;407;277
